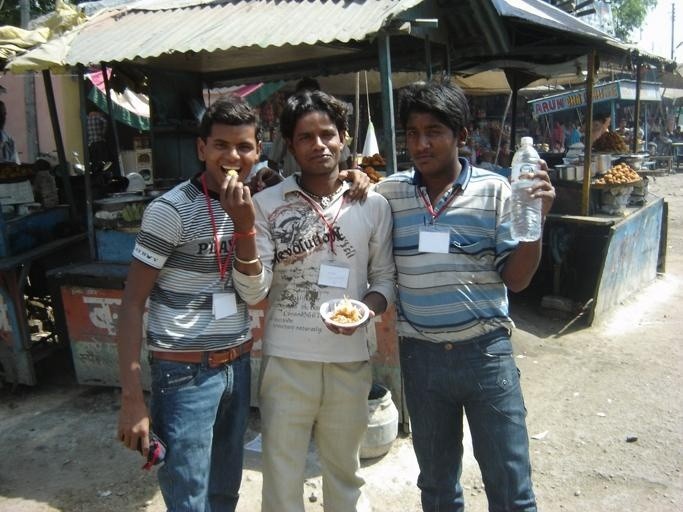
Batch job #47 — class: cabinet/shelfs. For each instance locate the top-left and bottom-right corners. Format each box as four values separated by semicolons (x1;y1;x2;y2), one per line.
120;147;153;187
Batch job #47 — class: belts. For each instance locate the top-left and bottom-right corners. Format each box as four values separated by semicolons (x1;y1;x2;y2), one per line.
153;337;253;368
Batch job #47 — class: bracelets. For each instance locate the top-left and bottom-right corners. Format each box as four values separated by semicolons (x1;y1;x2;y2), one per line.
233;254;260;265
231;230;256;239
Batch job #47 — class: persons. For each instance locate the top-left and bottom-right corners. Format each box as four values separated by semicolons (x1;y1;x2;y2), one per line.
219;89;398;512
249;79;556;512
267;79;350;177
460;118;658;165
0;101;20;165
87;108;108;160
117;96;370;512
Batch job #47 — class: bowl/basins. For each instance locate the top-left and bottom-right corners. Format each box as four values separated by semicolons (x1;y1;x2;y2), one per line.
557;152;611;183
18;202;40;218
318;298;370;333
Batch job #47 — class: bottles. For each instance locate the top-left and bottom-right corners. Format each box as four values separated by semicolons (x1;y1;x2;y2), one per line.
509;135;543;243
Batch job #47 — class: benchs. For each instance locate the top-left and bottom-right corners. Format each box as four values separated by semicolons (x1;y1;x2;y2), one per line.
627;141;683;185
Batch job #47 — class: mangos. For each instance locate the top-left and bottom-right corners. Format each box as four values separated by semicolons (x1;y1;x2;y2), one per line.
594;162;639;184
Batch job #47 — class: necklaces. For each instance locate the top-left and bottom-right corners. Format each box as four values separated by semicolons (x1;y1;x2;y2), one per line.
297;176;343;207
